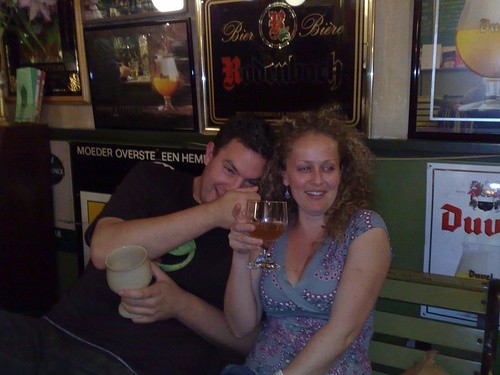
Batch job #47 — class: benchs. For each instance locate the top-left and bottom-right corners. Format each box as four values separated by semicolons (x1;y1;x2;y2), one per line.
371;266;500;375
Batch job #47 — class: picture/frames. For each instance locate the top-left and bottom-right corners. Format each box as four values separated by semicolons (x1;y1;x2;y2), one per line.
189;0;375;144
406;0;500;144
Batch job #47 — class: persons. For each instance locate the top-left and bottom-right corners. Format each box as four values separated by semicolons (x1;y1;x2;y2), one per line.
0;112;275;375
225;105;392;375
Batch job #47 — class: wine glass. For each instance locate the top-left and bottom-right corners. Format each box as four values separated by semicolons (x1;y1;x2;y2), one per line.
244;199;288;270
105;245;152;319
153;57;180;113
